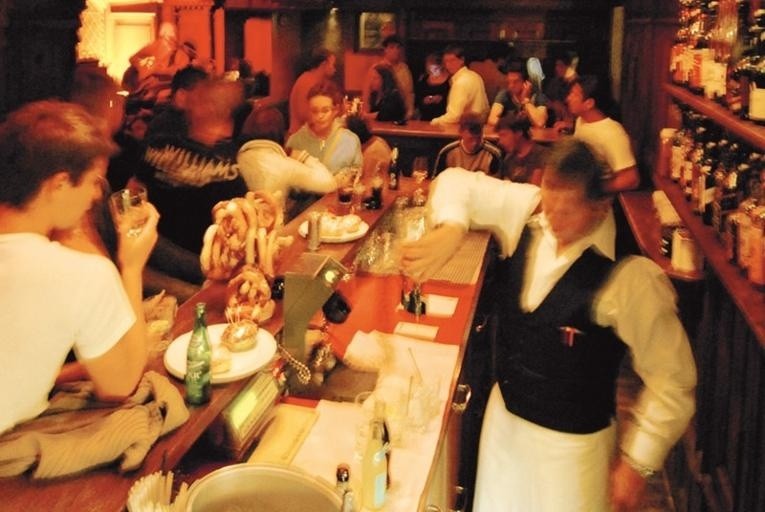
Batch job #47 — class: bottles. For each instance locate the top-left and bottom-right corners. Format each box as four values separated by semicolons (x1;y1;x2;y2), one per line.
668;0;765;131
183;302;212;406
388;148;402;189
373;408;391;492
658;101;764;301
399;206;428;300
366;425;388;511
368;164;382;207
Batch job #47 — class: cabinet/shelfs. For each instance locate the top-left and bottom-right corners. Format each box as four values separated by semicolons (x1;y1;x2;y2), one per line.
652;1;761;346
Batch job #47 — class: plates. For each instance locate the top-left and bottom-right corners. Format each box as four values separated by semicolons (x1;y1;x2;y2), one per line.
163;322;279;384
300;213;370;243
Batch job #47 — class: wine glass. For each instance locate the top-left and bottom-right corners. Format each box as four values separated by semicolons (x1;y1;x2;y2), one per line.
413;157;429;192
353;396;383;478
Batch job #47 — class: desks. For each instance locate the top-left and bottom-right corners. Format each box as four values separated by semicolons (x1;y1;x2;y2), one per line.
368;119;559;144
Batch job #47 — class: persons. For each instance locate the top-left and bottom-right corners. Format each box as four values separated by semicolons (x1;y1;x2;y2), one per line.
431;109;502;180
402;134;701;512
241;104;339;219
281;45;341;154
565;75;640;200
43;71;124;257
0;97;162;436
282;77;364;185
366;28;602;135
493;116;554;185
107;25;287;280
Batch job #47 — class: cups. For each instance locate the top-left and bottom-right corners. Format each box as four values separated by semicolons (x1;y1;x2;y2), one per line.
400;380;436;431
334;171;355;205
